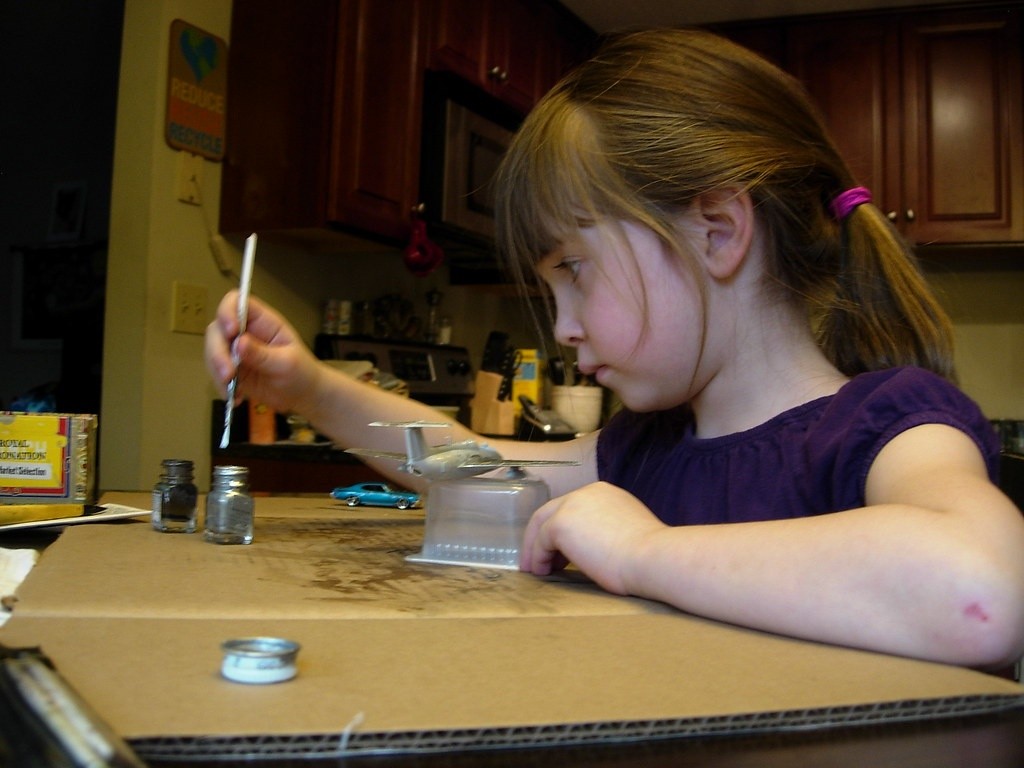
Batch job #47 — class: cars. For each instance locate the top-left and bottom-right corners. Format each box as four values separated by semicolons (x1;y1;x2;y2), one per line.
330;481;420;510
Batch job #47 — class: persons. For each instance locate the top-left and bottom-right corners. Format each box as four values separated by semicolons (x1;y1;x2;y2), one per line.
202;28;1024;690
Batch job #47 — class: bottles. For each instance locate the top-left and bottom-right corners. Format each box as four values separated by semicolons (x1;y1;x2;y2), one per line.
151;458;200;534
204;466;257;544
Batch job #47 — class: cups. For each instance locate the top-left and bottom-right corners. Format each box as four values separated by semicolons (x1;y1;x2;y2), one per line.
551;385;603;434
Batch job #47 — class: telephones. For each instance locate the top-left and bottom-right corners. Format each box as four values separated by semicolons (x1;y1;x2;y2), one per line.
516;393;579;441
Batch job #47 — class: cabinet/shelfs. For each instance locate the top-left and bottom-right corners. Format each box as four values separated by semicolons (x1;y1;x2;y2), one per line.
219;0;429;249
423;74;523;265
702;18;790;70
790;5;1024;269
430;0;601;125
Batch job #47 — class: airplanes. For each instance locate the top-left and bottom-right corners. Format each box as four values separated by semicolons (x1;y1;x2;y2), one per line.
344;422;583;482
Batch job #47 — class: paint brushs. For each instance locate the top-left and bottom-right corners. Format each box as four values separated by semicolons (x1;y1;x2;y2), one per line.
218;232;260;450
